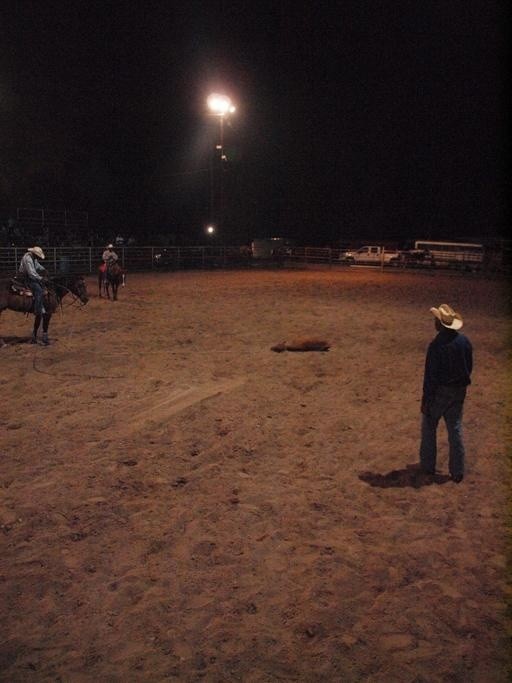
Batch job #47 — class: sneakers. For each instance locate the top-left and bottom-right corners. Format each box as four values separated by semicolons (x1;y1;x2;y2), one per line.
409;466;435;475
450;473;462;483
37;312;49;316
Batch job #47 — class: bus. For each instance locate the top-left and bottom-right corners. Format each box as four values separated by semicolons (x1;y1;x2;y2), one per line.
414;241;485;273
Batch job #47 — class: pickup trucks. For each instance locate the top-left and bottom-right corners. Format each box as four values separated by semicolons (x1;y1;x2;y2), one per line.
340;245;401;268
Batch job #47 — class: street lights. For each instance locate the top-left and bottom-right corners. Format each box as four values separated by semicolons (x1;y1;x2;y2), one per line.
204;91;236;241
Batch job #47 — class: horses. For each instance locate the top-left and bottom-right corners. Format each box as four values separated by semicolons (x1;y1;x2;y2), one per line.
98;268;128;301
0;273;90;348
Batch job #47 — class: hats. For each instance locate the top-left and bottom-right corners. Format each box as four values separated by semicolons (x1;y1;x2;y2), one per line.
105;243;114;249
28;244;46;259
429;303;464;329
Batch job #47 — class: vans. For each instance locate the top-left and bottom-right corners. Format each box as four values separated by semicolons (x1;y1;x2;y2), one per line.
240;236;295;261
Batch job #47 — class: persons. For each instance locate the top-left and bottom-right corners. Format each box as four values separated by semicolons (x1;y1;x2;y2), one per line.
406;304;473;482
15;246;47;314
2;224;294;284
